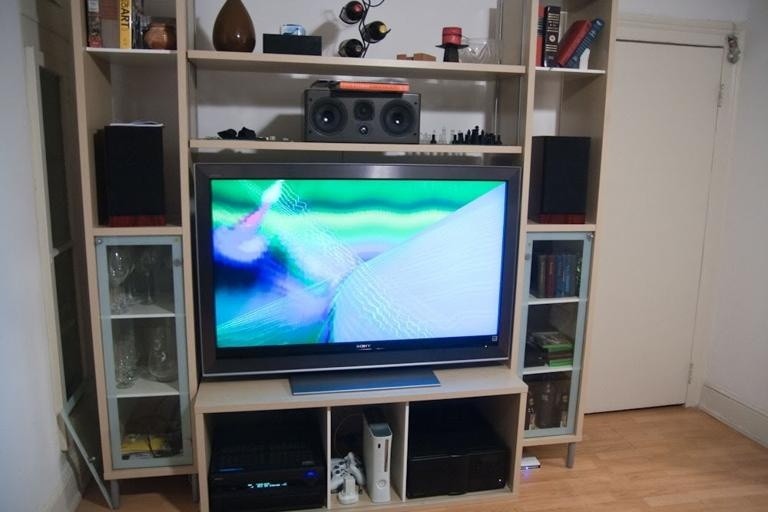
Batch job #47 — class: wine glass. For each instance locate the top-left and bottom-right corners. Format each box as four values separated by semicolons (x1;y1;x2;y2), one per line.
109;248;173;315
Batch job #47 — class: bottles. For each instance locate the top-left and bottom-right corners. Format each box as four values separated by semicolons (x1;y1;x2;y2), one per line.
116;333;177;388
525;383;569;429
212;0;256;53
143;23;175;49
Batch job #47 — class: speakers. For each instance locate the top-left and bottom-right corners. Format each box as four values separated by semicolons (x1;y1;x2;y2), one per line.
303;79;422;142
93;125;167;227
530;135;591;224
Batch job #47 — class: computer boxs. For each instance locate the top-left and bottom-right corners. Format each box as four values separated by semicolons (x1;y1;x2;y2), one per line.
408;402;512;497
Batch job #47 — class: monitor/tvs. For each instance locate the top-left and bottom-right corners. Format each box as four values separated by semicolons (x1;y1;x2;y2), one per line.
191;160;524;396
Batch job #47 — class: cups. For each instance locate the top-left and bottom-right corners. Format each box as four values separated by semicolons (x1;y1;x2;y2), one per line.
460;38;499;65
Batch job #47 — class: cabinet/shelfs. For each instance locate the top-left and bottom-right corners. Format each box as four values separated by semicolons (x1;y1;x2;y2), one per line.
195;365;529;508
71;0;620;510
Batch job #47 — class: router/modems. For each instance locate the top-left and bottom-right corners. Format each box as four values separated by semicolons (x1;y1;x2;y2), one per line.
521;454;540;469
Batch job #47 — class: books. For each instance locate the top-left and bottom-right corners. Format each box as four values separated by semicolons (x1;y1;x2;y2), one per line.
119;0;143;49
525;250;581;367
87;0;104;48
535;2;604;69
